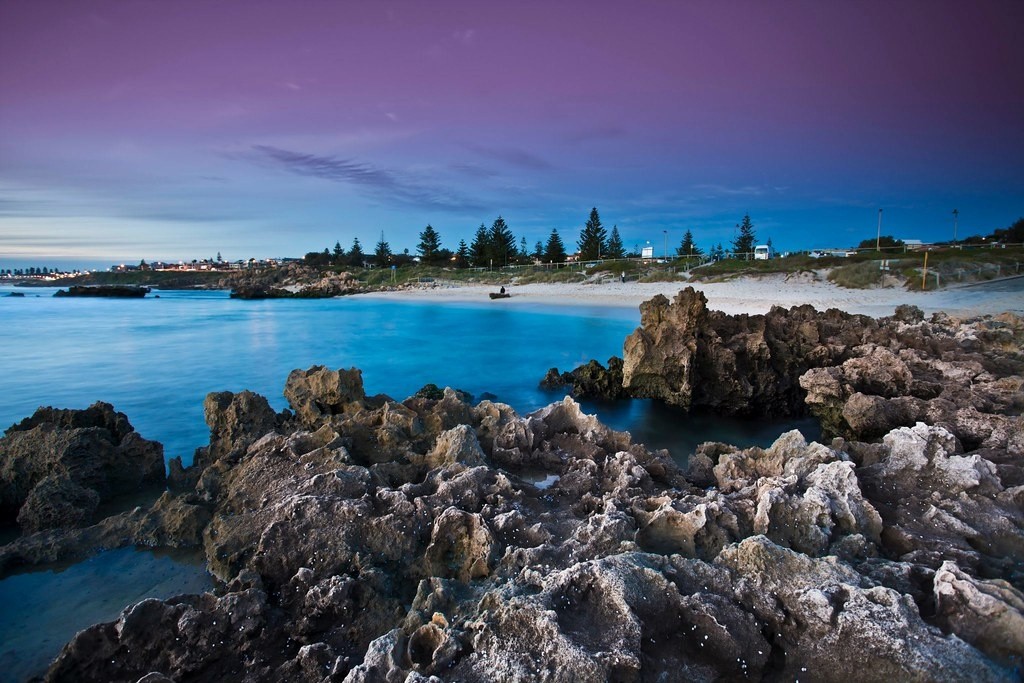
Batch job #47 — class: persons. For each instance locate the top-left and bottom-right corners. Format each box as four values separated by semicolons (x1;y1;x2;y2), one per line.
500;286;505;293
621;271;626;283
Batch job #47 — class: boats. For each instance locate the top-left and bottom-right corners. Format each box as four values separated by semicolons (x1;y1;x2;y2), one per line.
488;293;510;299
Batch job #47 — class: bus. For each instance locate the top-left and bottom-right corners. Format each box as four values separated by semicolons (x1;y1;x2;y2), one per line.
754;245;774;260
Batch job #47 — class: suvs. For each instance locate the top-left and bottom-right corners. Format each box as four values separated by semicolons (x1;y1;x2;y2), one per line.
586;260;605;270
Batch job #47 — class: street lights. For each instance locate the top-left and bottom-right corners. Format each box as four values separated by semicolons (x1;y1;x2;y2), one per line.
877;207;883;252
952;209;960;245
663;230;667;261
